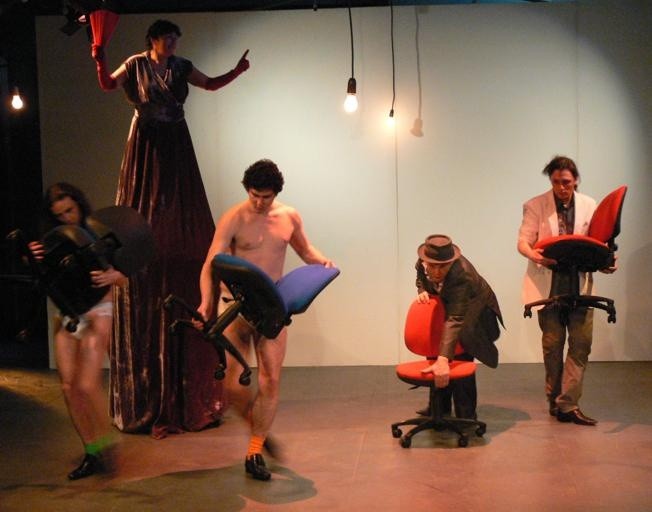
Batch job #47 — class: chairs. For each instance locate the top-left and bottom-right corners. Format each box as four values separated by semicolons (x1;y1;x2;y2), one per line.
391;288;488;451
521;182;631;326
2;202;159;347
164;258;344;388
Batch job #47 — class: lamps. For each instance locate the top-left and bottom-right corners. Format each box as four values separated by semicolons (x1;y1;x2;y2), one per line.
10;86;25;116
335;3;360;114
383;2;398;135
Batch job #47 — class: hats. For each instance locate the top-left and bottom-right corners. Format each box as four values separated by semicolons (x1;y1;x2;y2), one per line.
416;233;462;264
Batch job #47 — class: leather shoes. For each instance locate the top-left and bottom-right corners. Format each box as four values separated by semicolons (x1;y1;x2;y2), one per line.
244;453;271;482
415;406;429;416
68;453;96;479
263;437;284;460
548;398;559;416
94;447;114;476
556;408;599;425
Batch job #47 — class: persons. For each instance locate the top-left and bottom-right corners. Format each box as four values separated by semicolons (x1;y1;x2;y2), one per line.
22;182;129;483
190;159;338;479
91;10;250;264
516;154;620;425
413;233;507;430
